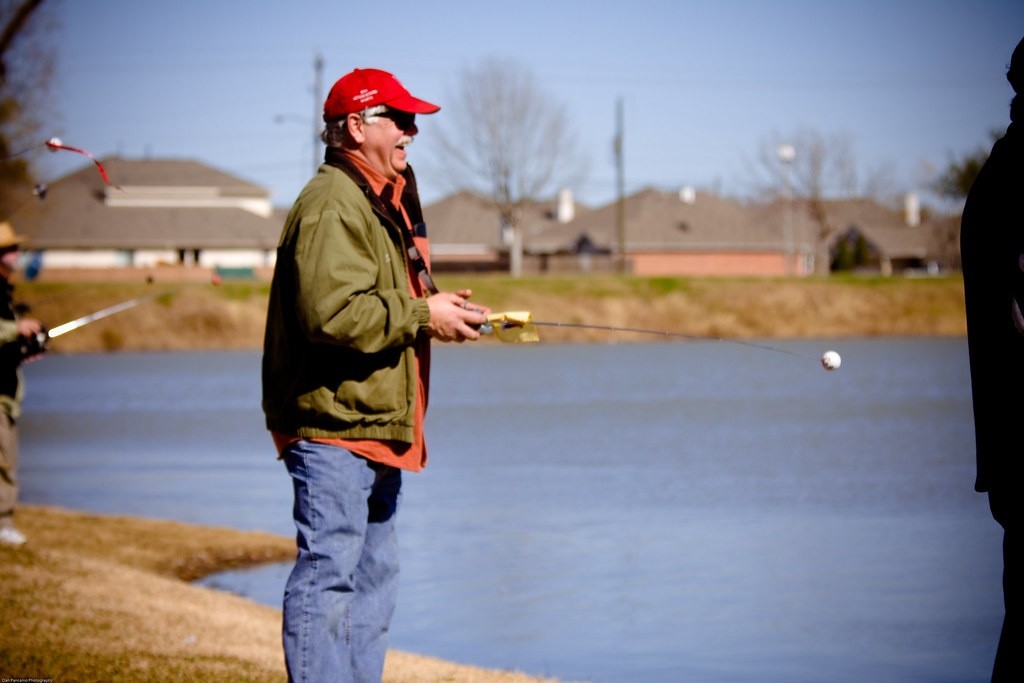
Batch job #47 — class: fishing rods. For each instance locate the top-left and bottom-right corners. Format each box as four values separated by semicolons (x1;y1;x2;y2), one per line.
0;134;62;173
33;273;226;351
480;304;843;371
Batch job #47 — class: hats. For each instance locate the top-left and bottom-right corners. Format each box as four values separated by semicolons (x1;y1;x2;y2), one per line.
323;68;440;122
0;222;28;248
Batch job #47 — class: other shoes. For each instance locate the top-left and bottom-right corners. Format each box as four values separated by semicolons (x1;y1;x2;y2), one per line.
1;529;28;545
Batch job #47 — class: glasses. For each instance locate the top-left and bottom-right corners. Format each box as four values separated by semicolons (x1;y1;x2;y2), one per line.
370;110;408;123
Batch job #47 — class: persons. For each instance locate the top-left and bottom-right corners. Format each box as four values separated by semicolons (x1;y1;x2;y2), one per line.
956;37;1024;681
260;69;493;683
0;225;41;547
